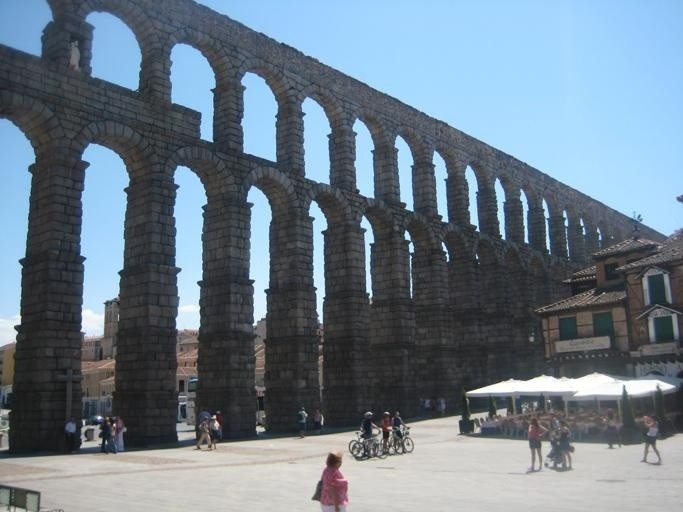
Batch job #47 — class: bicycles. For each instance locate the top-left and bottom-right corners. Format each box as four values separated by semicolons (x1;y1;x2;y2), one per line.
346;428;415;460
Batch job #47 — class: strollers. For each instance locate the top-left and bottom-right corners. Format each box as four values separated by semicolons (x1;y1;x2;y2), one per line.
543;442;562;471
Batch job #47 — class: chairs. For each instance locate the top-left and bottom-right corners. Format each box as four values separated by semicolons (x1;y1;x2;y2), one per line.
475;408;642;443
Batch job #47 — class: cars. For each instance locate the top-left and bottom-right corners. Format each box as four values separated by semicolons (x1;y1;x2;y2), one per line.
85;415;103;425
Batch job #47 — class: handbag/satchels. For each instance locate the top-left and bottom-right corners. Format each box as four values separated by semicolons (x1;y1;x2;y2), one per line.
311;480;323;501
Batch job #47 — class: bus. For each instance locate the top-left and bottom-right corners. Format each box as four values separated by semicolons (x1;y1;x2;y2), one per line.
185;378;198;424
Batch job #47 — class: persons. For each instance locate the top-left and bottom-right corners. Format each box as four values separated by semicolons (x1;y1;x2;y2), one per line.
208;414;219;451
214;410;224;441
296;406;308;438
390;410;411;455
98;415;127;454
196;415;212;449
311;407;324;434
358;412;381;455
63;416;77;452
525;407;663;472
381;412;393;454
318;450;349;512
419;395;446;414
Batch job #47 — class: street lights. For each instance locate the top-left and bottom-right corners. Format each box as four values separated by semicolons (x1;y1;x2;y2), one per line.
527;331;536;377
